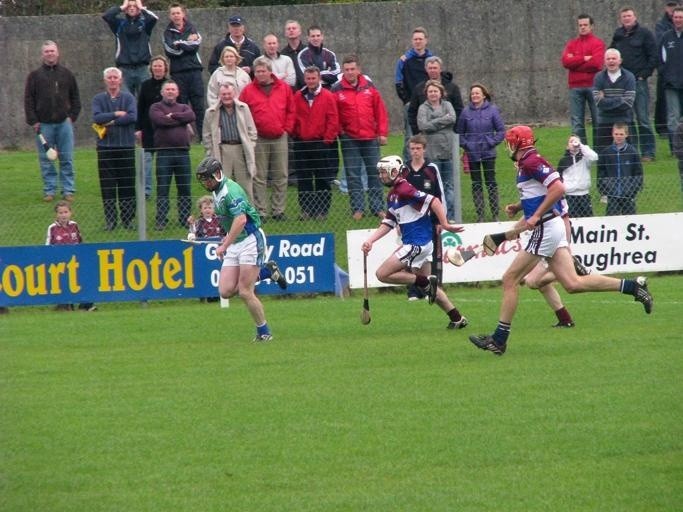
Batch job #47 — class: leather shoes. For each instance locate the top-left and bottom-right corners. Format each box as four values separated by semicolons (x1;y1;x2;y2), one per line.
154;218;166;232
182;217;193;230
477;215;484;223
315;212;327;222
641;158;651;161
66;195;75;201
275;212;287;222
353;210;363;221
105;221;118;230
493;213;499;222
123;221;134;230
260;215;266;223
44;195;55;202
376;211;386;219
299;212;310;221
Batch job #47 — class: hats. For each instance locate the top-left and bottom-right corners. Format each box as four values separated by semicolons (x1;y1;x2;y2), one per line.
229;16;243;25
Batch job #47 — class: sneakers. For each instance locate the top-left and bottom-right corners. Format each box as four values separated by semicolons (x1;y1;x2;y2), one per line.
447;316;468;330
408;295;418;301
552;320;575;328
633;275;652;314
424;275;437;305
267;260;287;289
250;333;273;342
572;256;589;276
468;335;506;355
424;295;429;301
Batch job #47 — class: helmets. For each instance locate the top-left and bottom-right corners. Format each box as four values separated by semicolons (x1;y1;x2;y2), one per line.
197;157;223;191
377;155;405;188
504;126;535;160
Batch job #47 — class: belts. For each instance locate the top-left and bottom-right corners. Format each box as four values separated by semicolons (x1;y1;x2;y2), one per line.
222;140;242;144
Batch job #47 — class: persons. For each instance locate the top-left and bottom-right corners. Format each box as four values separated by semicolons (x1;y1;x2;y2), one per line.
202;82;258;209
558;135;599;217
102;0;159;94
610;7;660;161
25;40;81;202
331;56;388;221
194;157;287;345
593;48;638;158
293;67;339;220
362;155;468;332
506;157;590;329
45;206;98;313
237;57;297;223
150;80;195;231
455;84;506;222
254;34;297;186
401;135;448;303
418;79;457;223
560;13;605;153
279;19;307;93
207;46;251;108
187;195;226;241
295;26;340;185
466;127;654;355
135;54;168;200
163;1;206;144
597;123;644;216
654;0;681;137
396;26;433;168
91;67;138;229
407;57;462;137
207;15;260;78
663;7;682;177
